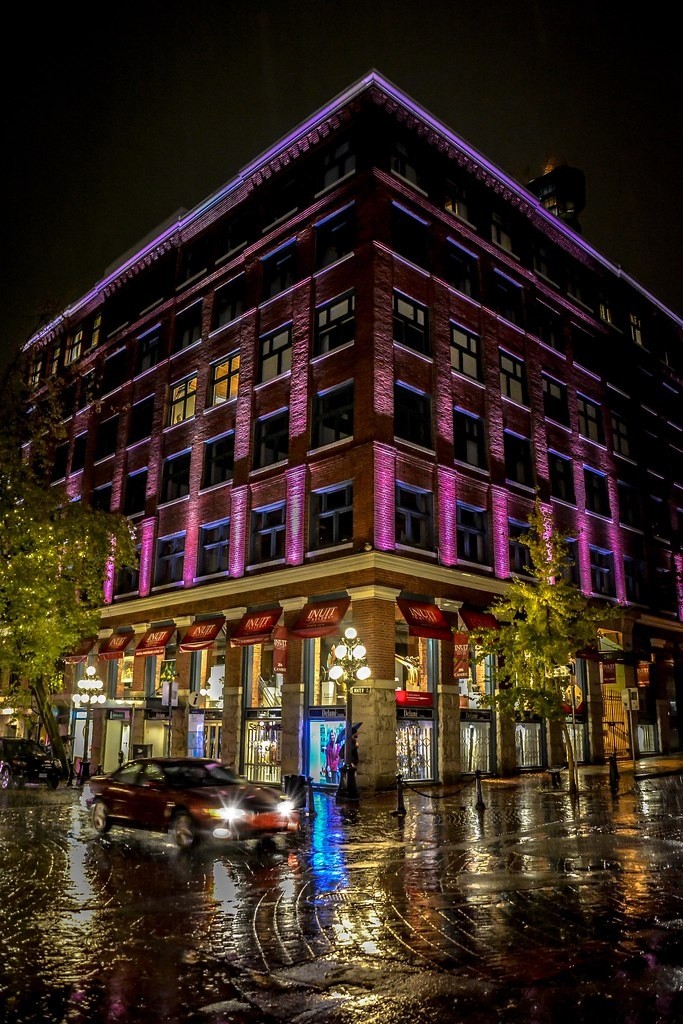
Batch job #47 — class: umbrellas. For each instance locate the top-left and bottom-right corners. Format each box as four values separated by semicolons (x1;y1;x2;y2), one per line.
59;735;76;748
335;722;364;744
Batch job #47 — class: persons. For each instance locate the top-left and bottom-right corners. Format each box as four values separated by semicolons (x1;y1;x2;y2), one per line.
336;728;360;796
64;738;75;786
323;729;341;782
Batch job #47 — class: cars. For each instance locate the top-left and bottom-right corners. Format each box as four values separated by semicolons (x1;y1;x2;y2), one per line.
84;755;305;849
0;736;63;790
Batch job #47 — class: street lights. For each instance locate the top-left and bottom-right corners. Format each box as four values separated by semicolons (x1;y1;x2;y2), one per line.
328;627;372;801
71;666;106;787
